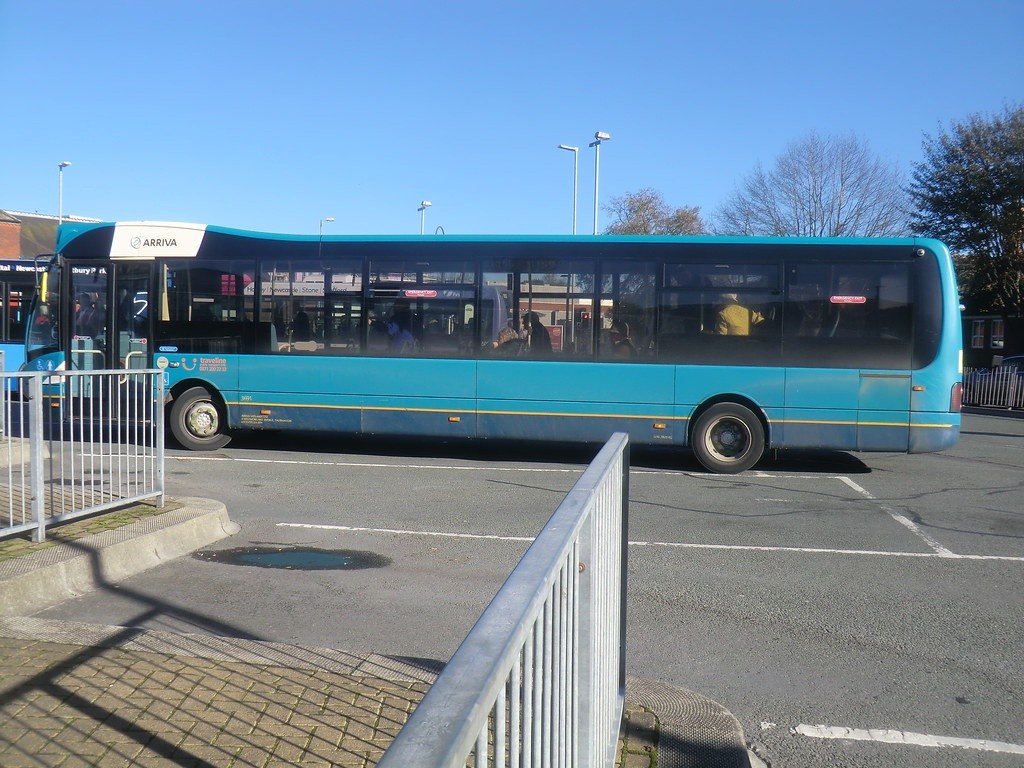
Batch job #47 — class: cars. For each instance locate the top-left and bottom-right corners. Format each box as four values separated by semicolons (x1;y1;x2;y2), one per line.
960;355;1024;410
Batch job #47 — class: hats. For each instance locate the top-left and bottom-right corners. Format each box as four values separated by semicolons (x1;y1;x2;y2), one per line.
719;294;738;302
521;312;538;318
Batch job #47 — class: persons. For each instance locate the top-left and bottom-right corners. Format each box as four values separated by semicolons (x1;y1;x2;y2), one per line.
673;267;765;337
521;313;552;357
72;294;98;332
370;299;425;355
608;322;636;359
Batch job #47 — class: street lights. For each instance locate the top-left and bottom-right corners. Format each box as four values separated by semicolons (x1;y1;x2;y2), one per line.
420;201;433;235
58;161;72;225
588;131;610;235
319;218;335;235
558;144;579;236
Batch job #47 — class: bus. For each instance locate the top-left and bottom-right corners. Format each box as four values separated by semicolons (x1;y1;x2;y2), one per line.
22;216;964;474
0;257;149;403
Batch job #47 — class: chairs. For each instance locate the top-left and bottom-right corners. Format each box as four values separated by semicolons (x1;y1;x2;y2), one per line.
660;303;843;337
